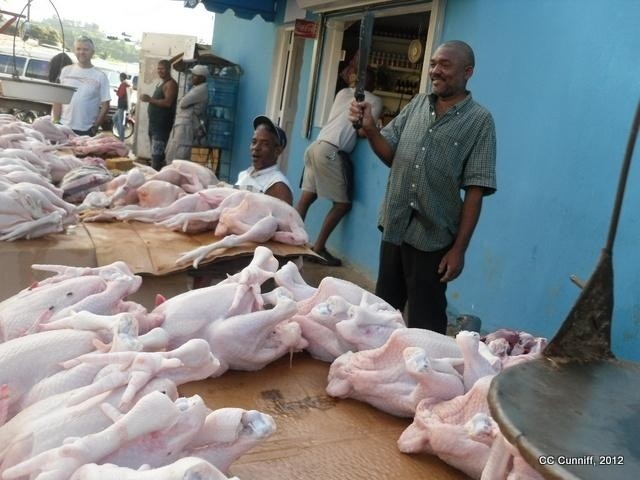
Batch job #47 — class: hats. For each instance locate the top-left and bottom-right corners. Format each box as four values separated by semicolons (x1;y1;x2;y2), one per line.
253;114;287;148
189;65;209;76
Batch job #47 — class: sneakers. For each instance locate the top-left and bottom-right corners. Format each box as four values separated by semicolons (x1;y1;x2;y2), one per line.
306;247;341;266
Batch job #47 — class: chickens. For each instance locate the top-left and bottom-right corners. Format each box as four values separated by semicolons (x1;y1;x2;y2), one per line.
274;260;548;480
0;113;308;480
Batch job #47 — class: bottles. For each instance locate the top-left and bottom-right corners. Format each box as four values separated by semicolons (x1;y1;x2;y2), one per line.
393;75;419;94
369;49;423;71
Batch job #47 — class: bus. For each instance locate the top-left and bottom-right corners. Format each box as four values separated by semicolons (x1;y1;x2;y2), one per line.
116;61;140;116
0;32;134;132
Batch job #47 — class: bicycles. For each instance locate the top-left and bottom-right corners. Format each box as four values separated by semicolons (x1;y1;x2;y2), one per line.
110;102;136;140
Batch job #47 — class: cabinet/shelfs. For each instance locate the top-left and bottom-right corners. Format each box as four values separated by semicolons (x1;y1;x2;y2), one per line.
369;35;425;102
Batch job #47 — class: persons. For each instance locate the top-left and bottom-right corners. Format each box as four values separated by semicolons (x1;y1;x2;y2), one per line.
188;114;305;290
294;70;384;265
50;34;113;138
114;72;130;142
139;59;179;171
347;40;495;335
163;64;212;168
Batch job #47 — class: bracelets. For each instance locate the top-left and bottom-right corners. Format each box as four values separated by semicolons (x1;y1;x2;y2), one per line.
92;122;98;131
53;117;60;124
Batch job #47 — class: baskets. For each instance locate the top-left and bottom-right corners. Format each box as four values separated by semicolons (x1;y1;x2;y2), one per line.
187;76;240;151
